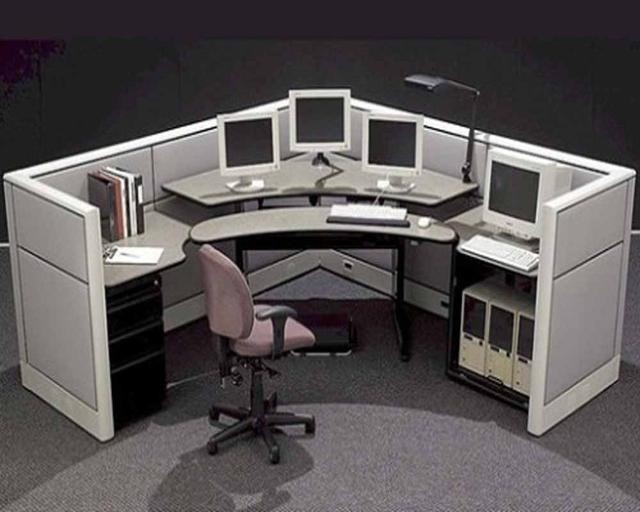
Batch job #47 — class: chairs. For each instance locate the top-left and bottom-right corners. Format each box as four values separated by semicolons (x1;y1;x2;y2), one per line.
198;241;316;464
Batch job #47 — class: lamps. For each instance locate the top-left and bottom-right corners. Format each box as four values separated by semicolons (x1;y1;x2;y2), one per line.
402;72;481;183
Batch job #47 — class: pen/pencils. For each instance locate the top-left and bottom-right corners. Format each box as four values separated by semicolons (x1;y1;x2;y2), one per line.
120;252;142;260
